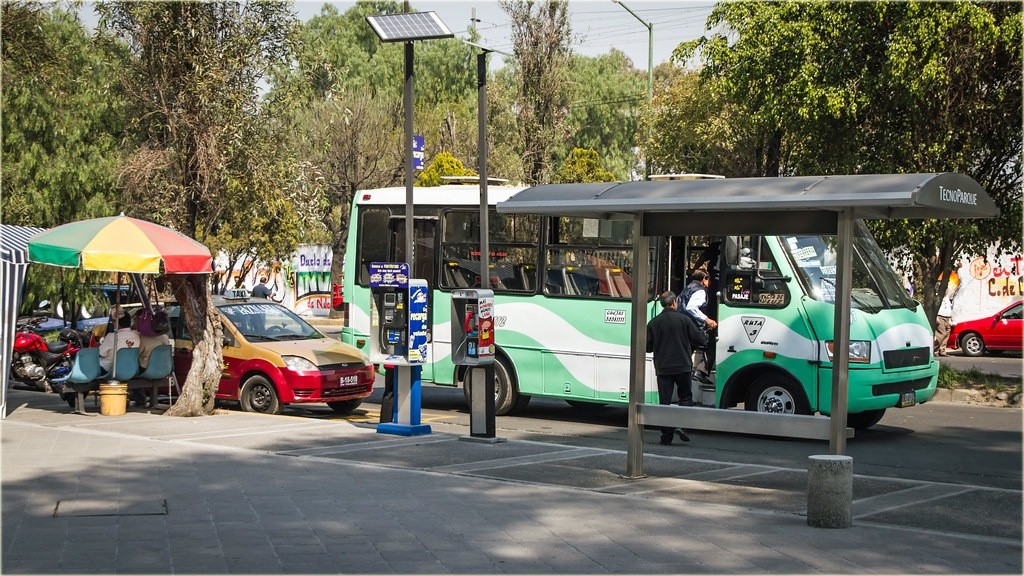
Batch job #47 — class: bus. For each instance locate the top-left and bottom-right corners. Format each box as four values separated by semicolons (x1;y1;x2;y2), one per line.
341;175;940;416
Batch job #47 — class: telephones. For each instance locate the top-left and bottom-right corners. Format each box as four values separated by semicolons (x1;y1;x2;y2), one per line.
382;293;408;328
464;304;479;337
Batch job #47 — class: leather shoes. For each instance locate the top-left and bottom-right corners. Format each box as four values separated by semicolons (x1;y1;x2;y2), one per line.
691;370;713;385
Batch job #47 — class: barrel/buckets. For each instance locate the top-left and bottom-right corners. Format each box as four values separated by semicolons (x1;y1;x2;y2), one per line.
100;383;128;416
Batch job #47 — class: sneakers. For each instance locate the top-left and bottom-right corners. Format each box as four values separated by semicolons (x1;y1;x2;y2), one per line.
674;428;690;442
659;441;671;446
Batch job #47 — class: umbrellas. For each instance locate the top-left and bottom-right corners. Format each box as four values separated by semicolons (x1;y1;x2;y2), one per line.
28;212;216;381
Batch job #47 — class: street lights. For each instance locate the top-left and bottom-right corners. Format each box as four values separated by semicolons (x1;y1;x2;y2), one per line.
613;0;653;181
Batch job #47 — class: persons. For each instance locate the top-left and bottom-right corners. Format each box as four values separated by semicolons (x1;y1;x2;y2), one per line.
132;312;171;407
229;275;247;330
60;311;141;408
673;269;717;384
933;285;953;356
250;277;276;332
105;298;131;337
646;290;706;447
685;241;720;347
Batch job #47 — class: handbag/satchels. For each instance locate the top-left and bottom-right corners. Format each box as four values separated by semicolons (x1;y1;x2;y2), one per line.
137;279;169;337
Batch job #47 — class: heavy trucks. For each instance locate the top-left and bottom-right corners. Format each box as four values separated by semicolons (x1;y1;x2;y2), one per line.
22;283;130;321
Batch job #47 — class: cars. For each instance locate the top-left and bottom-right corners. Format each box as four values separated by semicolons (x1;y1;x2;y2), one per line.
89;289;375;415
946;300;1024;356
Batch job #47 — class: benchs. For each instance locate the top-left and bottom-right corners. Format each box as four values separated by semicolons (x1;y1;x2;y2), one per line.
51;344;175;416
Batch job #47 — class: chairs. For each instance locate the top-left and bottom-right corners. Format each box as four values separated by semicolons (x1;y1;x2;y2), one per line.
187;318;265;347
444;261;470;288
509;261;633;298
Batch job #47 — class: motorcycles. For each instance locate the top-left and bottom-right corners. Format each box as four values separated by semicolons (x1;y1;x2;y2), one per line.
10;299;97;394
